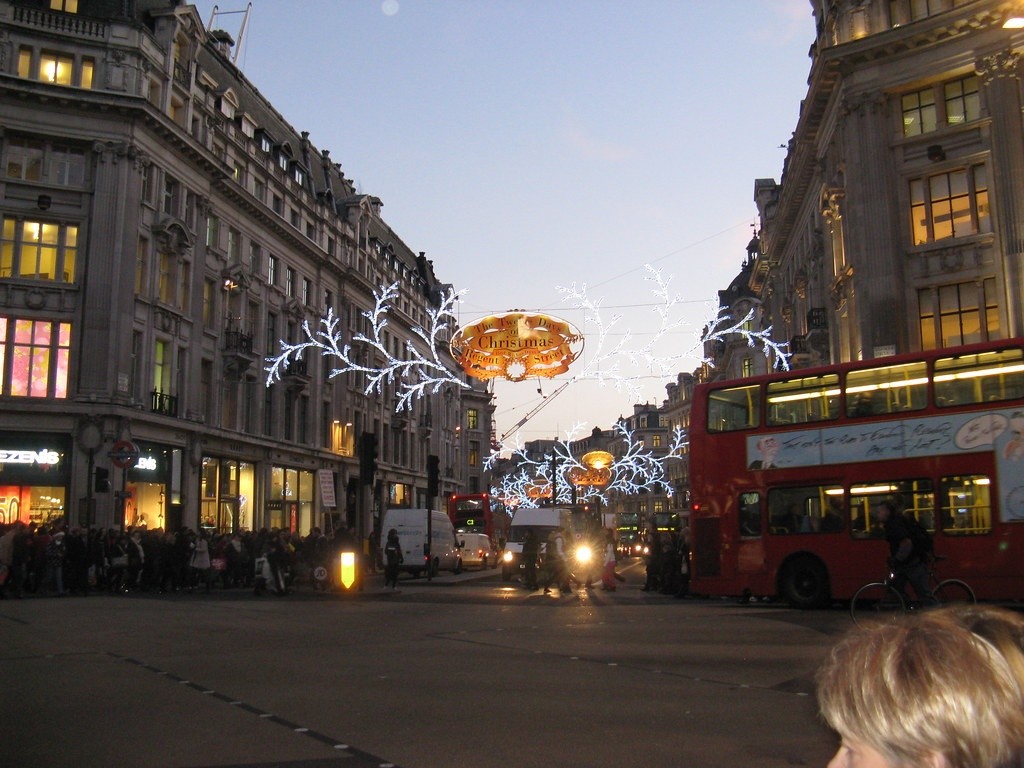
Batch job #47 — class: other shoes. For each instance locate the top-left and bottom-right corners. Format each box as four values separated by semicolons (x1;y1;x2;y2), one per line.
639;586;649;592
254;588;295;598
98;585;181;599
907;600;922;612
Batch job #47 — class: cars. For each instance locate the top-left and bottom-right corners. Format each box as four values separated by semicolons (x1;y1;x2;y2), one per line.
453;531;496;568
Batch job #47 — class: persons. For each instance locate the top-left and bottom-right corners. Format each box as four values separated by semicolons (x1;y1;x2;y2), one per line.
640;527;690;595
740;501;877;534
522;528;618;593
0;518;405;600
876;500;935;608
811;605;1024;768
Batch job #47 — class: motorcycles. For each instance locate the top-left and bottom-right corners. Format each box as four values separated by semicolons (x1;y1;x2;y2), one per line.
247;543;290;601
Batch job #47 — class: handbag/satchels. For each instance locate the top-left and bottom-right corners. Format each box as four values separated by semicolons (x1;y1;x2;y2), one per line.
255;554;270;579
110;544;129;568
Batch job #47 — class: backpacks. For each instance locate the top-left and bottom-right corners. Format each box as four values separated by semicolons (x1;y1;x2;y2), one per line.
896;513;932;562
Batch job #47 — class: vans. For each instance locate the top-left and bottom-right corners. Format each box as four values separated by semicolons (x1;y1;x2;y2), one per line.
500;508;576;585
377;507;463;577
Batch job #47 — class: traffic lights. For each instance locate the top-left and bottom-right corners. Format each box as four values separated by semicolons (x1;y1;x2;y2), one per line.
427;455;440;501
356;433;380;486
94;465;111;493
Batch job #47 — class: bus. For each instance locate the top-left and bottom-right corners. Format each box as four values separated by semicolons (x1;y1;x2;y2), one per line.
687;338;1024;610
449;492;508;556
617;513;640;557
653;512;690;559
539;498;616;570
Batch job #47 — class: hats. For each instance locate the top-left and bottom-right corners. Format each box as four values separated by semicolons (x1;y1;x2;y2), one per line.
52;527;65;538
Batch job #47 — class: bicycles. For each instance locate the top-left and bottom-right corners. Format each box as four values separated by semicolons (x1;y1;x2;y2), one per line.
847;560;977;632
